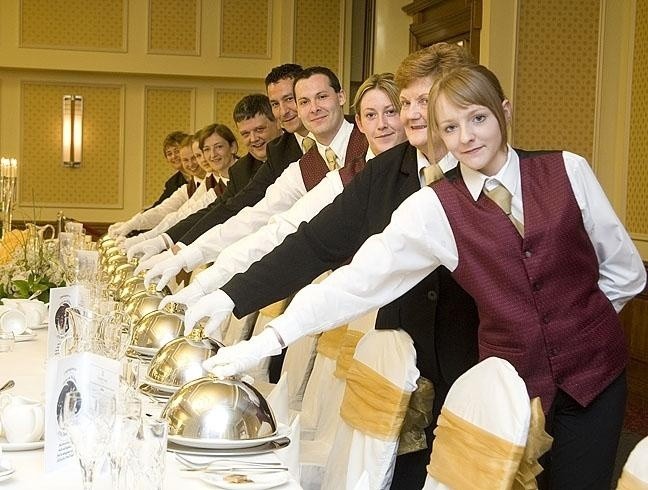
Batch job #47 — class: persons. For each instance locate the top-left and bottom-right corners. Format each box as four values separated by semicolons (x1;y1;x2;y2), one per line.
203;65;648;490
158;71;408;310
184;43;478;490
106;66;369;291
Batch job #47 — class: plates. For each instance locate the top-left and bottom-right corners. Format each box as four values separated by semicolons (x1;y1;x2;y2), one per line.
130;344;160;359
162;421;292;451
199;471;289;488
0;335;38;342
1;442;47;452
139;374;182;396
0;470;16;481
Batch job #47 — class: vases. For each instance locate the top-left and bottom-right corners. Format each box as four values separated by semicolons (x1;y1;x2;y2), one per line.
1;297;49;330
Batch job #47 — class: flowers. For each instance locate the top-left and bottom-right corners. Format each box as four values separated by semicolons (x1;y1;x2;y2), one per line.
0;187;67;301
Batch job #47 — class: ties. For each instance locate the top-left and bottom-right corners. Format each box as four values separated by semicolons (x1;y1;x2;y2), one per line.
487;185;524;239
325;148;340;170
302;138;316;152
420;164;443;186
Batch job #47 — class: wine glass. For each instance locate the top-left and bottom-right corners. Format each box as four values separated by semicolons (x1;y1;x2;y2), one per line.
65;390;143;490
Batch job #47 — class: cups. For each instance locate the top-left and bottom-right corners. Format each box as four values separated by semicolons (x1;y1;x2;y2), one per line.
59;222;100;287
0;329;15;354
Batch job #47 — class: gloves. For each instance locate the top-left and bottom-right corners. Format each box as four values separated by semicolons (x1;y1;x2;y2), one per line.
158;279;235;337
202;327;282;380
108;212;187;291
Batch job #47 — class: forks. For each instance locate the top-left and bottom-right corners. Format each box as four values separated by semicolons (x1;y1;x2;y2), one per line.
169;455;283;471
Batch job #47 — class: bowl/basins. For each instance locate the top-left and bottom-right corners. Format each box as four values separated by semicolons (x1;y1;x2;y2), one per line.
1;311;28;337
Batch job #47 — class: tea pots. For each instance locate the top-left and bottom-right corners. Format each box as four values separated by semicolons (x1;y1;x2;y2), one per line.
65;307;133;356
0;391;45;442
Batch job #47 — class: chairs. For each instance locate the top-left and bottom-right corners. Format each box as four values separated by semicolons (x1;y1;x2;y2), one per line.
613;434;648;490
214;267;379;490
419;353;554;490
320;325;434;490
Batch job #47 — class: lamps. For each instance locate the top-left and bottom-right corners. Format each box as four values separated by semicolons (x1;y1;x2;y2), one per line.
62;94;85;169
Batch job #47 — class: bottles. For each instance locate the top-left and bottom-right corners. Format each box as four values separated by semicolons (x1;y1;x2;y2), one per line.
24;223;56;241
